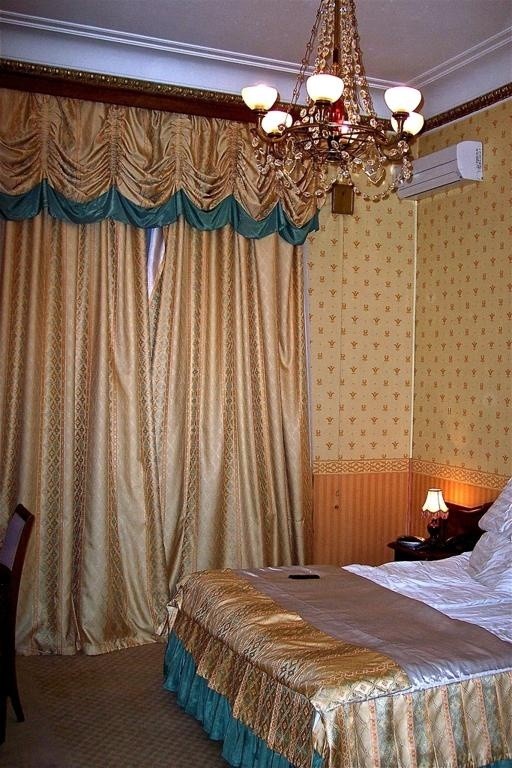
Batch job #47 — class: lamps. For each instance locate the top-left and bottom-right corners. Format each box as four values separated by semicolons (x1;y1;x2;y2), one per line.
241;0;425;201
421;488;449;549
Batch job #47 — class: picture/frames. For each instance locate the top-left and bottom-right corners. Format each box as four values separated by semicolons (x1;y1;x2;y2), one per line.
332;183;354;214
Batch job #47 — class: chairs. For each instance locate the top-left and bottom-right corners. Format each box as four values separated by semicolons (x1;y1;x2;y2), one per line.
0;503;35;722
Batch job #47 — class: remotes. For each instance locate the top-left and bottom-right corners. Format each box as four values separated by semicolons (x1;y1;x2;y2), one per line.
288;573;322;580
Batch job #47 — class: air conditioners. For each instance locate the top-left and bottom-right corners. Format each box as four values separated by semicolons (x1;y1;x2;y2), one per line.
396;141;483;199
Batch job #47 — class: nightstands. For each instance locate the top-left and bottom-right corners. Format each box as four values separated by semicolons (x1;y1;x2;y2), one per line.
387;535;458;561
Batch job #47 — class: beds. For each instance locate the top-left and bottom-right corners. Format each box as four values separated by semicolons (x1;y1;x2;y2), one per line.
161;500;512;768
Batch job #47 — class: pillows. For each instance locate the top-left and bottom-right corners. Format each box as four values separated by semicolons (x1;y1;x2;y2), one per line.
467;530;512;594
478;477;512;538
